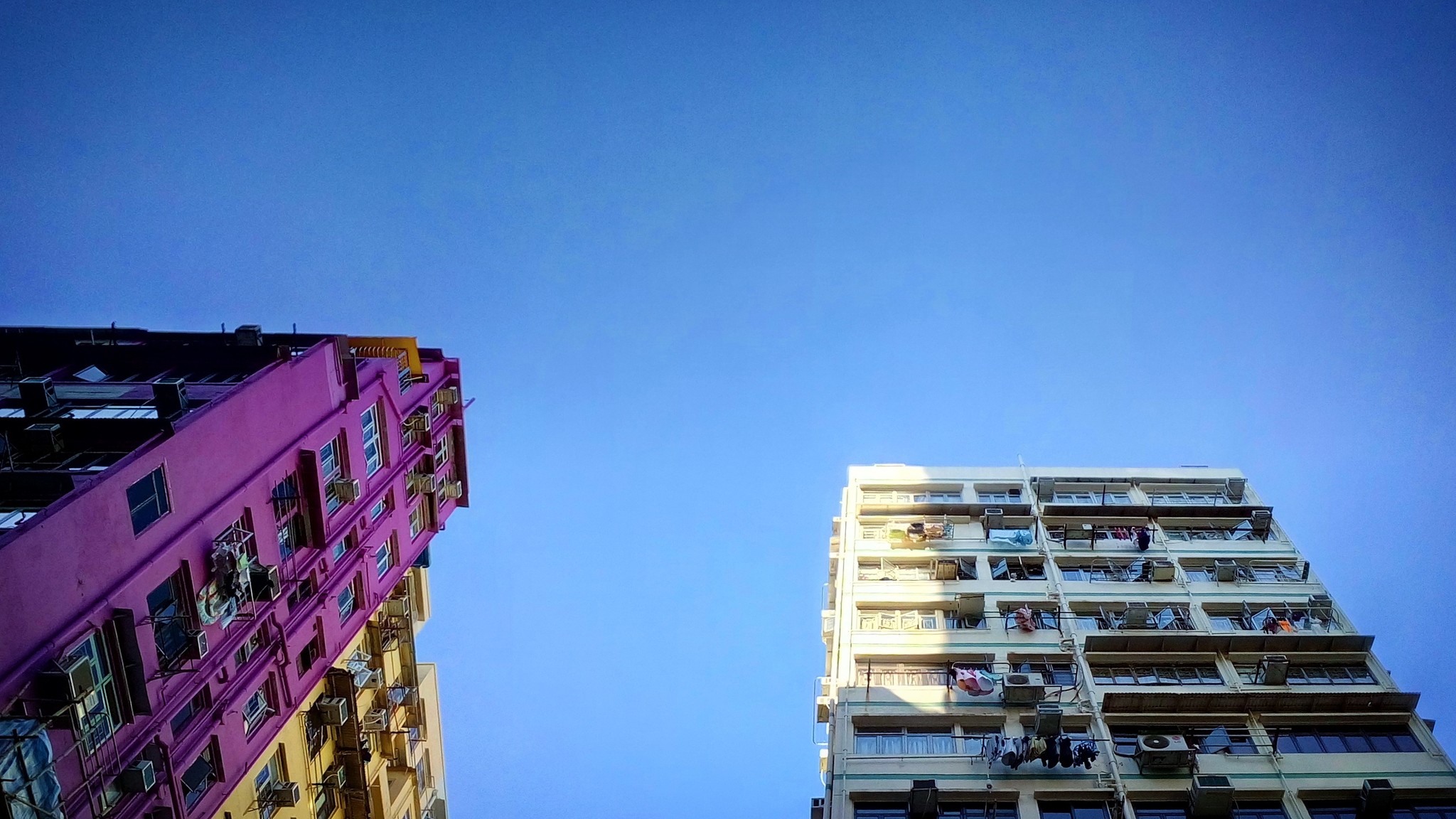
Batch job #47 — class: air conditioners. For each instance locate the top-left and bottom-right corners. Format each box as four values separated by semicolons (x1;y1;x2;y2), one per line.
1;325;461;819
809;477;1394;819
